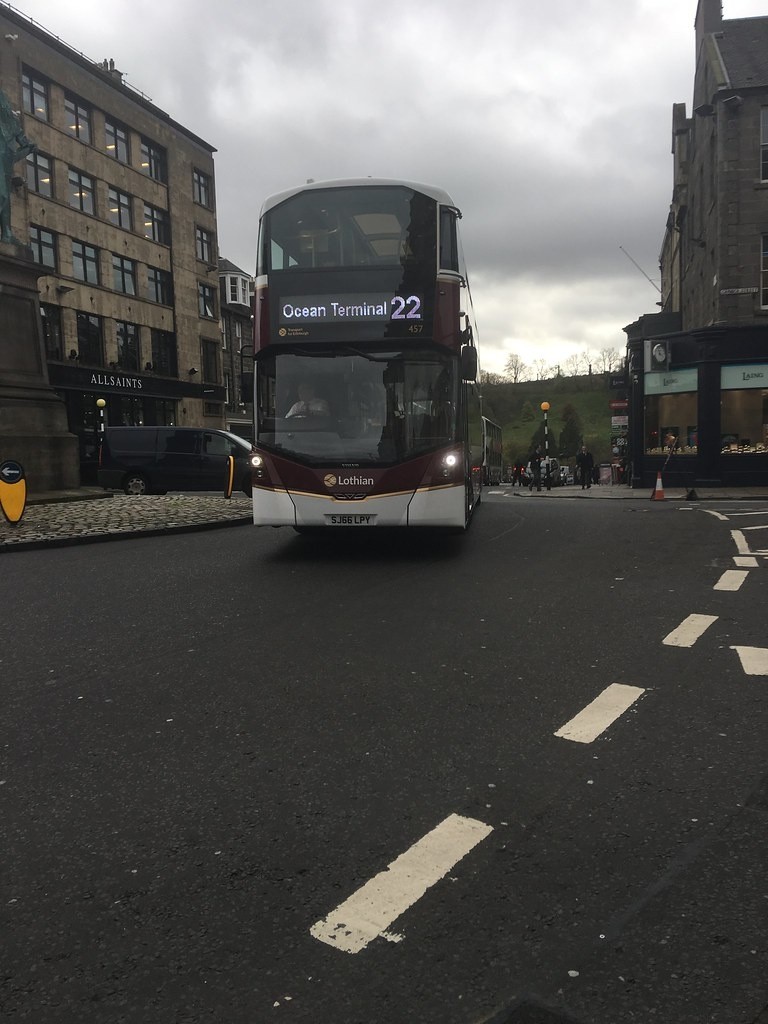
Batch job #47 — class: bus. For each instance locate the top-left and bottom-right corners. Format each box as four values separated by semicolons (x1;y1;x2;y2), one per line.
479;401;502;485
239;176;484;531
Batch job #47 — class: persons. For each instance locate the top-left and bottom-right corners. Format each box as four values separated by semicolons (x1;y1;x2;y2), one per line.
285;380;333;418
576;445;593;490
570;466;577;482
593;464;600;485
528;444;545;491
510;461;523;487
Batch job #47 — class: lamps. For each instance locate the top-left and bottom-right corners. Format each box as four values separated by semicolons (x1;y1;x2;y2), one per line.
189;367;198;375
145;362;152;371
205;265;218;272
694;104;715;117
723;96;743;108
69;349;77;360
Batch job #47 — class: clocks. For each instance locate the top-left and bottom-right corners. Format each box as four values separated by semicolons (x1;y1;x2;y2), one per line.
653;344;666;362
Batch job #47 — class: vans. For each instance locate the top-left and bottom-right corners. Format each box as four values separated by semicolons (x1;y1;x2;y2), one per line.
99;426;253;497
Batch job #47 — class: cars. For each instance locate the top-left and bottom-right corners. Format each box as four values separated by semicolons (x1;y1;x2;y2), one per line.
505;457;621;488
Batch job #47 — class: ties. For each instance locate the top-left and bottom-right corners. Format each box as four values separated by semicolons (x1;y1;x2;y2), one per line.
304;402;310;410
584;453;585;456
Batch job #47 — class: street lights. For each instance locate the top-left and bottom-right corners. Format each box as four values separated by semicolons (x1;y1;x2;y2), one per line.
96;398;105;488
541;402;550;491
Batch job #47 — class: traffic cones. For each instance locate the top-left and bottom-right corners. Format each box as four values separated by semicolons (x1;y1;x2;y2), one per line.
652;472;670;500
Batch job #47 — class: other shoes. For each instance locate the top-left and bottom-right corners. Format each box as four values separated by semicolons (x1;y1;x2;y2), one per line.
582;486;584;489
586;484;591;489
528;484;532;491
536;488;542;491
